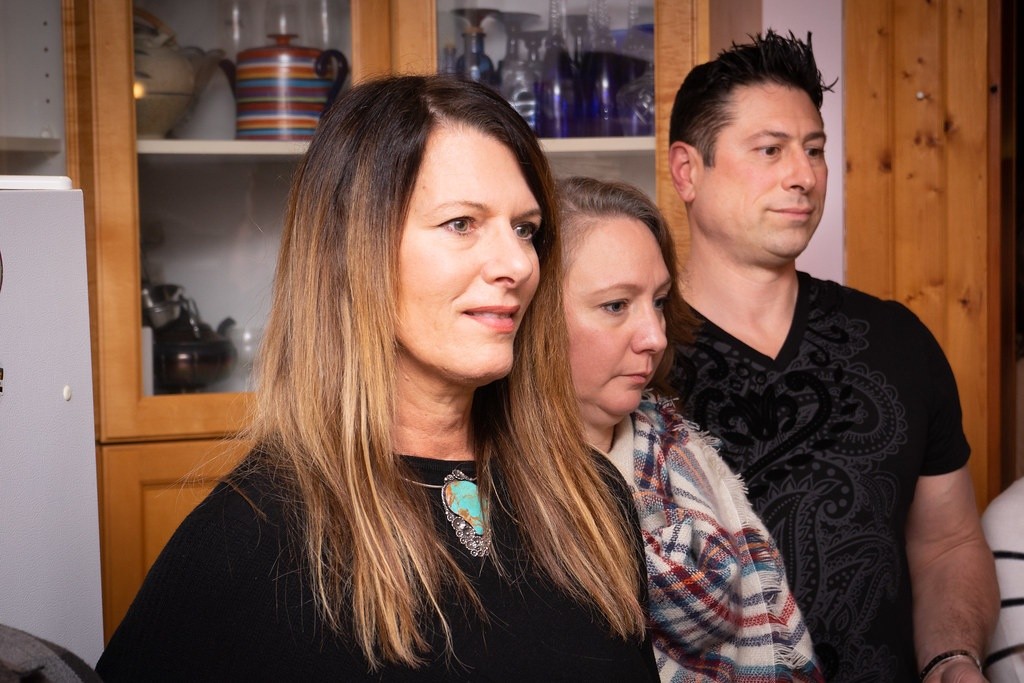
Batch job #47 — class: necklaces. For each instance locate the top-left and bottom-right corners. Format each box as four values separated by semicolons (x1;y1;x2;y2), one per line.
393;440;496;556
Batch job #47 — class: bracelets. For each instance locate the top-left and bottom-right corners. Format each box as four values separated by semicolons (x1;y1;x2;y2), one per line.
917;647;983;683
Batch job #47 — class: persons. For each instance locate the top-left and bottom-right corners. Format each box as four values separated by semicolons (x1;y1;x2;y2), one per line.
89;70;663;683
552;176;826;683
981;475;1024;683
647;20;1001;683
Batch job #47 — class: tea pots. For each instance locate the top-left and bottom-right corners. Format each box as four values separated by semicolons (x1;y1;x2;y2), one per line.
150;288;241;392
133;5;226;140
218;28;347;141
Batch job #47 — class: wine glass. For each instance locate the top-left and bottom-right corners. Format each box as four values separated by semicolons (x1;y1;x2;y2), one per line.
429;1;655;140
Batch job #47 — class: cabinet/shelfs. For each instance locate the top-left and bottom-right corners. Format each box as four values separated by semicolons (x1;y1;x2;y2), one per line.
63;1;765;651
